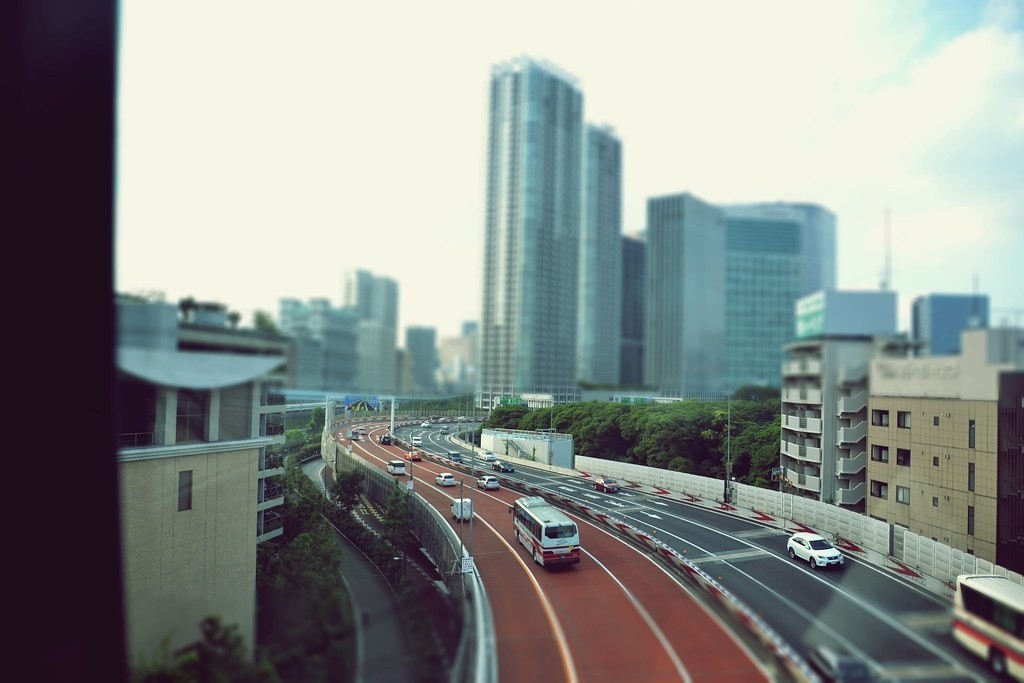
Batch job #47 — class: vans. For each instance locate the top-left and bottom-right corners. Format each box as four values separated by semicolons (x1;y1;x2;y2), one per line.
410;436;422;447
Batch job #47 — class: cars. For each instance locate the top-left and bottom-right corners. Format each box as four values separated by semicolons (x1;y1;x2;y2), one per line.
378;434;391;445
421;420;432;428
435;472;456;487
593;476;620;494
358;426;366;435
492;459;514;472
439;426;449;434
404;449;422;462
346;429;359;440
478;449;496;461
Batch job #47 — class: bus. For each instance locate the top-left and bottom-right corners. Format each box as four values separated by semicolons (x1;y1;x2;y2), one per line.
951;573;1024;682
509;495;580;568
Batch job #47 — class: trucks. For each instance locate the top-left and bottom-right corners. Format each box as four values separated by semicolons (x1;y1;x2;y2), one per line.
450;498;474;523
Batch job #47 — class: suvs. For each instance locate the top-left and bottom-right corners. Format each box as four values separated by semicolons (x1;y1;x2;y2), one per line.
448;450;463;463
476;475;499;490
386;460;406;475
786;531;845;569
